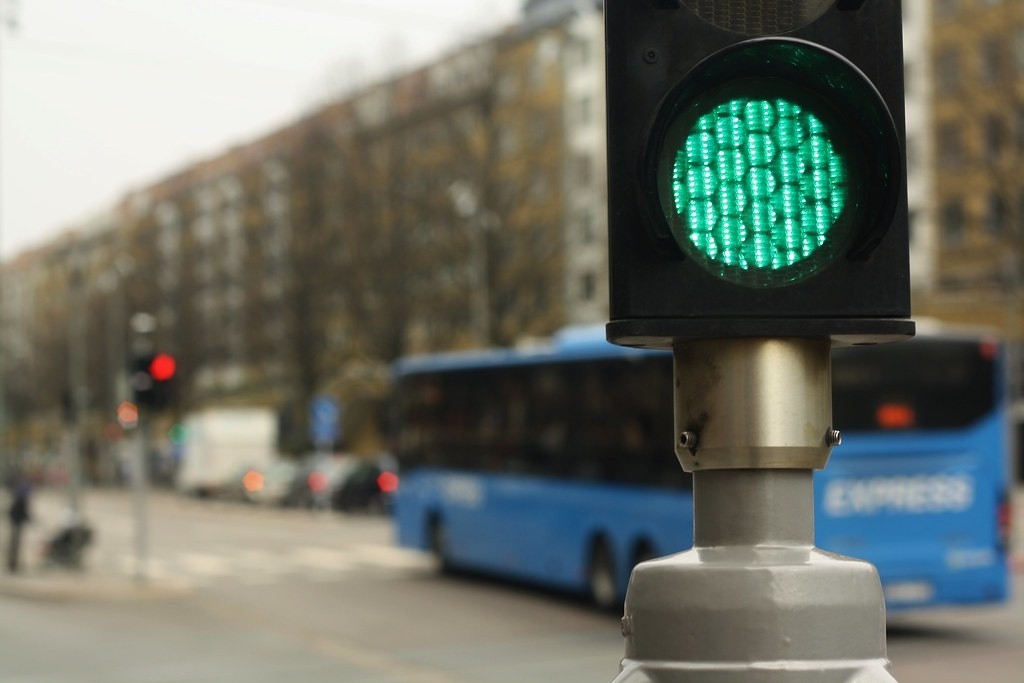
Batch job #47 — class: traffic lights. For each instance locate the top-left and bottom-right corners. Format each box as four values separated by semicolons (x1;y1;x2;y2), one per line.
149;352;177;383
639;0;900;299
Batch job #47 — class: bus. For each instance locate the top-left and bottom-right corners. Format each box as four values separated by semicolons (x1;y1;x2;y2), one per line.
386;315;1013;620
